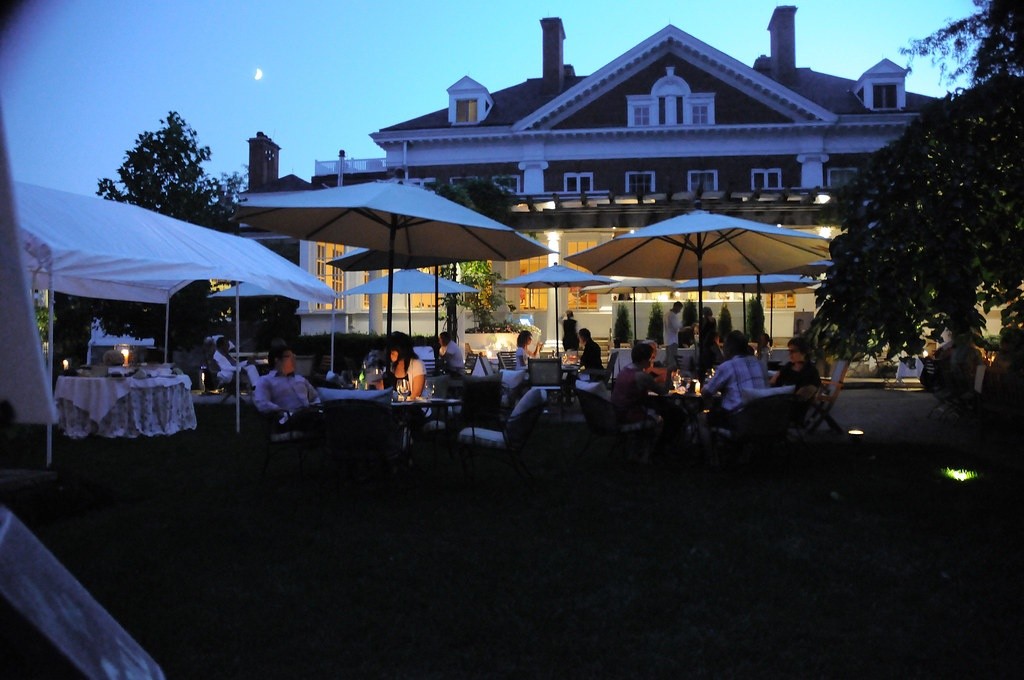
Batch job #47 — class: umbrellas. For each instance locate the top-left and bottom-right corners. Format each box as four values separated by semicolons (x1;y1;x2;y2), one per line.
207;184;835;377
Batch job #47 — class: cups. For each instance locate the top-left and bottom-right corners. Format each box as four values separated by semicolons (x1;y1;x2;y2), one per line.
114;344;129;366
548;355;552;359
341;370;352;383
670;371;700;394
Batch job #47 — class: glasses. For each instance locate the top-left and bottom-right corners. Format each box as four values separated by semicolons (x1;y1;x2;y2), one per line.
283;353;297;360
787;349;800;355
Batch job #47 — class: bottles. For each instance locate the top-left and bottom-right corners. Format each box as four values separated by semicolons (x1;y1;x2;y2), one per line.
358;360;367;390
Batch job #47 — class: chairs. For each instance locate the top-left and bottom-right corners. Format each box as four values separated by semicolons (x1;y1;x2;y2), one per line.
208;328;1024;505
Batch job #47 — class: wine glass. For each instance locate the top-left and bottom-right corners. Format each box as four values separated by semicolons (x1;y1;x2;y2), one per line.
396;379;435;403
562;354;567;364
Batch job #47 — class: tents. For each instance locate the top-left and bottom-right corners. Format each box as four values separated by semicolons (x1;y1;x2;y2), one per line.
13;180;337;471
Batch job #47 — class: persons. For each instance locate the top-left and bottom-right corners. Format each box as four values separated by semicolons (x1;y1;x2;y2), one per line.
213;302;1024;476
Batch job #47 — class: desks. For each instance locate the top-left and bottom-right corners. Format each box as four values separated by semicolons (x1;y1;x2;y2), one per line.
561;363;580;406
390;396;464;475
648;389;720;466
54;374;197;440
